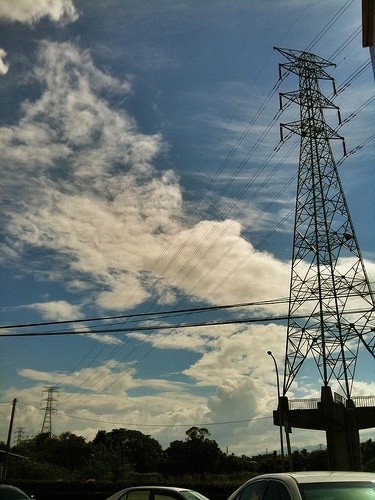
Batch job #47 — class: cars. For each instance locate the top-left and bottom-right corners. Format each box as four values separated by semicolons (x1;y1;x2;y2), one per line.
105;486;211;500
229;471;375;500
